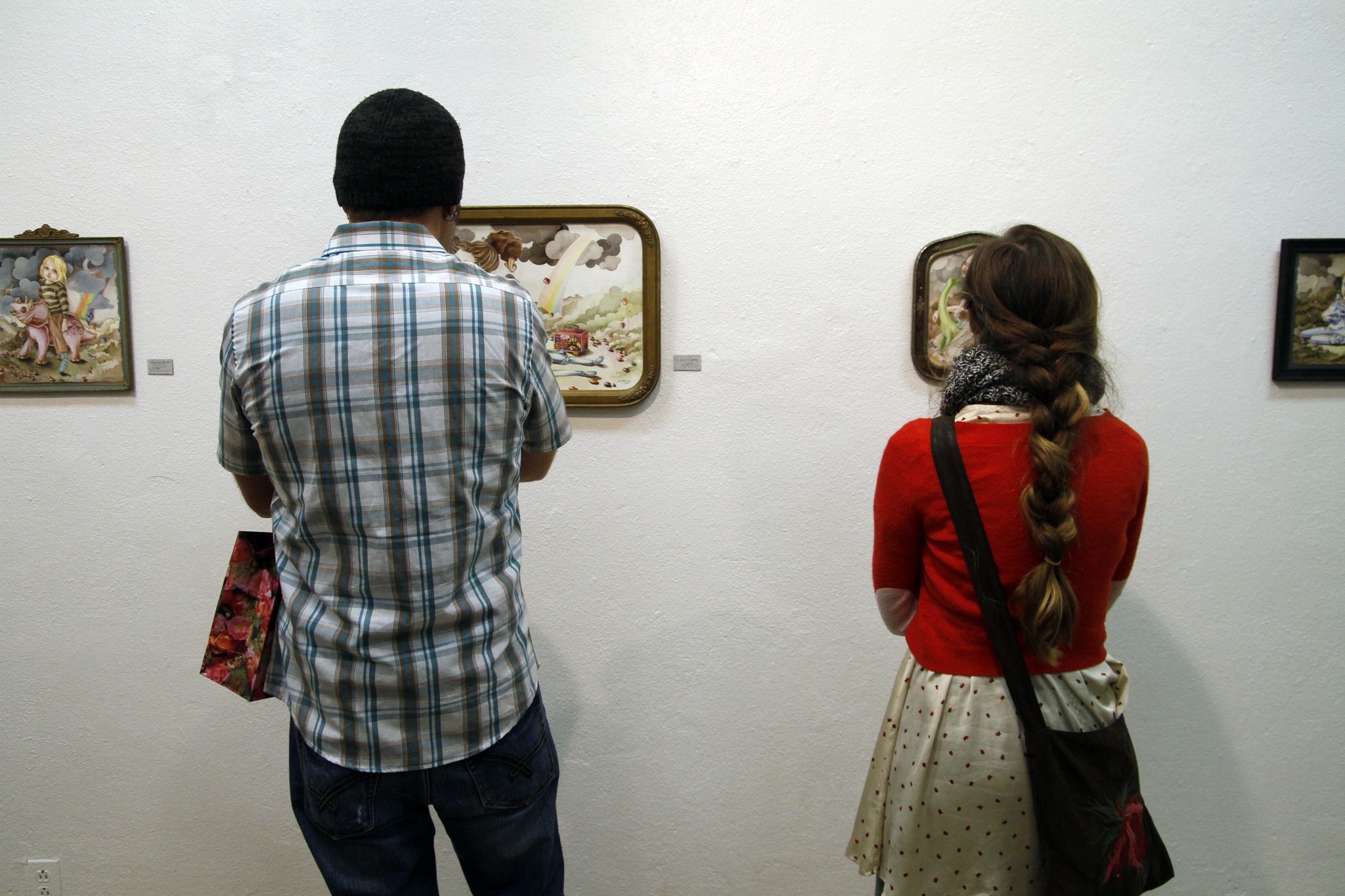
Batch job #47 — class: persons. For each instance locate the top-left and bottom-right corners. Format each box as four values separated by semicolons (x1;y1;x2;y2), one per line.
217;86;573;896
838;220;1152;896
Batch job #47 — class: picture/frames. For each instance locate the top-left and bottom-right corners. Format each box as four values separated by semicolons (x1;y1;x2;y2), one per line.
0;221;135;395
1268;236;1345;386
445;201;666;411
909;229;1006;394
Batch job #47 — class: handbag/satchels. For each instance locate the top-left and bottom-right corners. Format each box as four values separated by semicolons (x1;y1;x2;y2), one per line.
187;520;287;703
1020;713;1177;896
